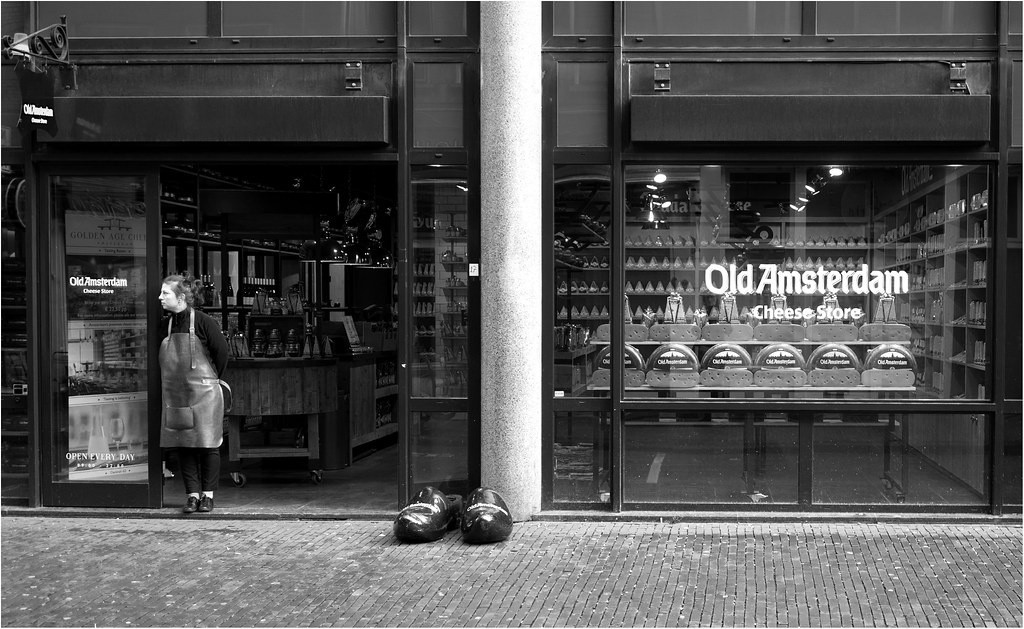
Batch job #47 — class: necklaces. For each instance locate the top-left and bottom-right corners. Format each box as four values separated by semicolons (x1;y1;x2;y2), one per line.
774;300;784;324
669;299;680;324
826;301;837;324
881;300;892;324
722;299;734;324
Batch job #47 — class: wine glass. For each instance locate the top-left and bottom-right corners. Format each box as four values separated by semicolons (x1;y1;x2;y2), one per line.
108;417;125;457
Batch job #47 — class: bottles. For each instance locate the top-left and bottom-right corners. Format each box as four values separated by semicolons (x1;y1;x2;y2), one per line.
241;276;277;307
198;273;216;306
912;306;925;322
226;276;234;307
915;371;924;387
912;276;926;291
916;243;926;259
169;271;184;276
912;336;925;355
88;406;109;460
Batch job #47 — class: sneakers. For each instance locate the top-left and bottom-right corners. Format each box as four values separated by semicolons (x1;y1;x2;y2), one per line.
182;496;199;512
199;495;214;512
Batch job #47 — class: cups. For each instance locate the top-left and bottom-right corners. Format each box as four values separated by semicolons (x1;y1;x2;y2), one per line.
127;438;143;455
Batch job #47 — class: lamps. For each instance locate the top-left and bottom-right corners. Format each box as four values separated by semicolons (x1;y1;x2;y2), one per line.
798;195;809;203
790;202;799;211
804;180;816;193
652;197;662;206
661;196;671;208
651;190;660;199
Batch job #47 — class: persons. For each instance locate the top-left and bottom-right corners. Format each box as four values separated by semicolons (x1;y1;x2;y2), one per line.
157;274;229;513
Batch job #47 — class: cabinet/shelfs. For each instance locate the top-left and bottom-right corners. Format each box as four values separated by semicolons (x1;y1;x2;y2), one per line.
586;339;916;503
393;248;435;338
440;235;470;397
160;163;307;334
872;165;989;499
557;243;901;429
1;305;30;500
